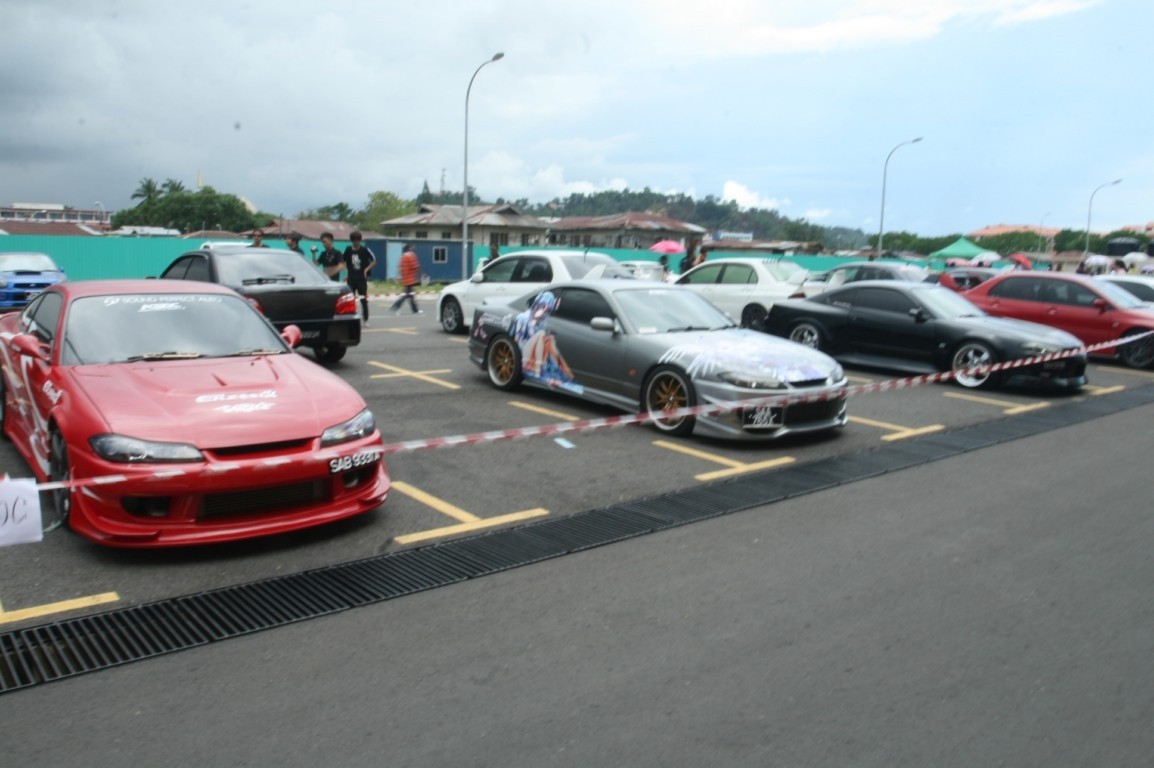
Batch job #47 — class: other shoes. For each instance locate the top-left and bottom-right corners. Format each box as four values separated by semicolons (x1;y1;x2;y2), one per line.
363;322;369;328
413;310;424;315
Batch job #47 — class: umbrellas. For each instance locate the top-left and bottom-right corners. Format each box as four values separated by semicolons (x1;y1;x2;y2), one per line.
1009;254;1032;270
649;240;686;254
1122;252;1150;263
929;237;1002;268
1084;255;1109;265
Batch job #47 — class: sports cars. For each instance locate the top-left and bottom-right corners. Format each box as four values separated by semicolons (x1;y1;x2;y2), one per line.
0;279;391;554
762;280;1091;394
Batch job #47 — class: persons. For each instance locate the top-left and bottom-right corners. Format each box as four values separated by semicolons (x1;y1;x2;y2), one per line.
343;230;378;327
946;263;955;269
483;240;499;266
977;261;983;267
817;247;824;255
680;246;708;274
311;232;345;282
869;254;874;260
247;228;270;248
1075;260;1139;275
390;245;423;314
659;255;669;282
1047;262;1063;270
286;232;304;255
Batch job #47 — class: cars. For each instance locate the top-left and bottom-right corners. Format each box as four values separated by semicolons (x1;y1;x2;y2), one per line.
141;245;363;365
0;251;69;311
580;261;679;292
664;256;812;326
800;259;1154;368
437;248;635;334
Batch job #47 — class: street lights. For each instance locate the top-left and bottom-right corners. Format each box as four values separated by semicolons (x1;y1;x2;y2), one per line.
1082;171;1126;271
460;47;509;286
873;132;927;265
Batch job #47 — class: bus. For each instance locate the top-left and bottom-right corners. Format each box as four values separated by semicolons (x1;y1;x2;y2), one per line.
468;278;853;443
